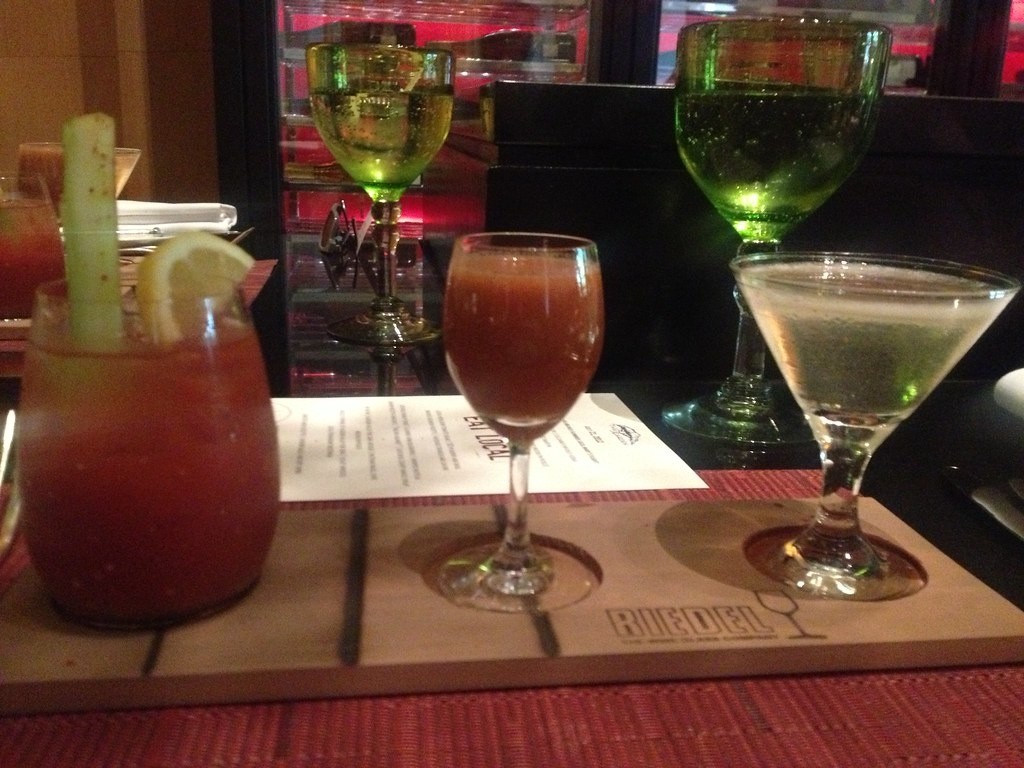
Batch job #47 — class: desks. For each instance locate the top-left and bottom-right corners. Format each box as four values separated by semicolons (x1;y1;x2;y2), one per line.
0;250;1023;768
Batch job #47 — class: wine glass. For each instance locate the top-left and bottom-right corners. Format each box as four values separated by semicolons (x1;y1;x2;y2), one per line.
435;231;605;612
307;43;457;344
662;18;893;445
729;251;1021;603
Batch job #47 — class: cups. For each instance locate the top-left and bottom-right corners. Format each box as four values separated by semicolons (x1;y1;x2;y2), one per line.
1;172;67;320
20;279;281;631
19;142;140;219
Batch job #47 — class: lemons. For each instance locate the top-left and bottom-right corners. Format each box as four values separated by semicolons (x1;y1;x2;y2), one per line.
137;231;255;348
